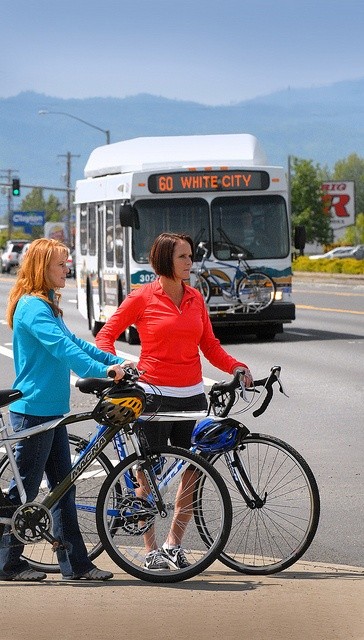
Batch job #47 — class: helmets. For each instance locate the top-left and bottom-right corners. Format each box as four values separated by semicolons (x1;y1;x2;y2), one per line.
192;418;250;454
90;382;146;427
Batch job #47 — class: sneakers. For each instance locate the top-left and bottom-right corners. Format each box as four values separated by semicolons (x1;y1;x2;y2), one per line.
64;563;114;581
143;550;169;572
161;545;192;570
1;566;47;581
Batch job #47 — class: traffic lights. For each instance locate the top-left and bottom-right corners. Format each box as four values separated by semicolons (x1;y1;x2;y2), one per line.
12;179;20;196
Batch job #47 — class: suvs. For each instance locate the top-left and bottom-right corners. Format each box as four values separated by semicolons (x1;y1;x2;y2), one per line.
0;239;33;274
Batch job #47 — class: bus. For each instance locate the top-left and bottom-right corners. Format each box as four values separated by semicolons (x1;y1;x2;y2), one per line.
73;133;296;346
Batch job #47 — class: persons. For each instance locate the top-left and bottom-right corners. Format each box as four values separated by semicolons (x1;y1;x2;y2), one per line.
92;232;252;571
0;238;135;583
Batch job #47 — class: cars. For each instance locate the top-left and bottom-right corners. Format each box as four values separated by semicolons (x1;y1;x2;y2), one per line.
308;246;356;261
16;242;76;278
330;244;364;260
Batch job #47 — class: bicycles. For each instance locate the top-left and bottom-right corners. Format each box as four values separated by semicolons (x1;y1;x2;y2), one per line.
188;240;276;315
0;363;321;575
0;364;234;584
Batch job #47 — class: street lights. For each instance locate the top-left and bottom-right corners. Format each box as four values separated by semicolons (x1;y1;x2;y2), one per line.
37;109;110;145
